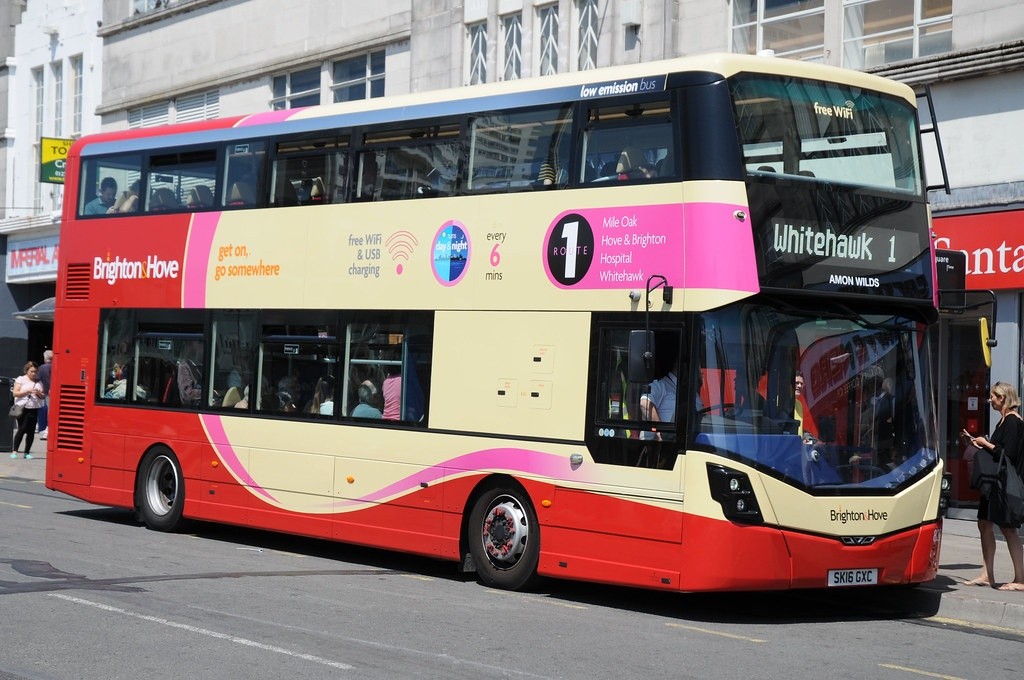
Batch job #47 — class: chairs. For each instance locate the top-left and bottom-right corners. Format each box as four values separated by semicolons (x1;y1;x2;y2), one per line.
122;145;646;212
222;387;241;408
625;370;658;423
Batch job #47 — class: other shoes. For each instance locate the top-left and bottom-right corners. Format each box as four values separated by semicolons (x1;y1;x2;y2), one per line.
10;454;18;459
23;453;33;459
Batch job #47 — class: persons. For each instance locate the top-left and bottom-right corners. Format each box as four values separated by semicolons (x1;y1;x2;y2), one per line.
85;177;120;214
861;377;895;469
38;350;53;440
794;371;804;440
10;362;44;459
105;338;403;421
733;361;768;426
640;353;704;441
963;382;1024;591
538;131;569;186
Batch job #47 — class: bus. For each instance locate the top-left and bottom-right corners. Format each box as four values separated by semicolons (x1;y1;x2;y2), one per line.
42;52;998;602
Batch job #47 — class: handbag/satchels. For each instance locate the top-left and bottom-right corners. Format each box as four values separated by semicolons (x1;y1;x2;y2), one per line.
987;448;1024;528
8;403;25;419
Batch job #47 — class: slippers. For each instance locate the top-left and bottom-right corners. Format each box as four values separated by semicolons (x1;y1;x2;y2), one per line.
998;583;1024;591
964;579;995;587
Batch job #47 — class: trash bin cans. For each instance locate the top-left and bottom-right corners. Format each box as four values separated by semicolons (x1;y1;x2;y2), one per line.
0;376;15;452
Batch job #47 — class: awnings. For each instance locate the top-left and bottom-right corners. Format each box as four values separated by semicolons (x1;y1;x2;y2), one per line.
12;298;54;322
